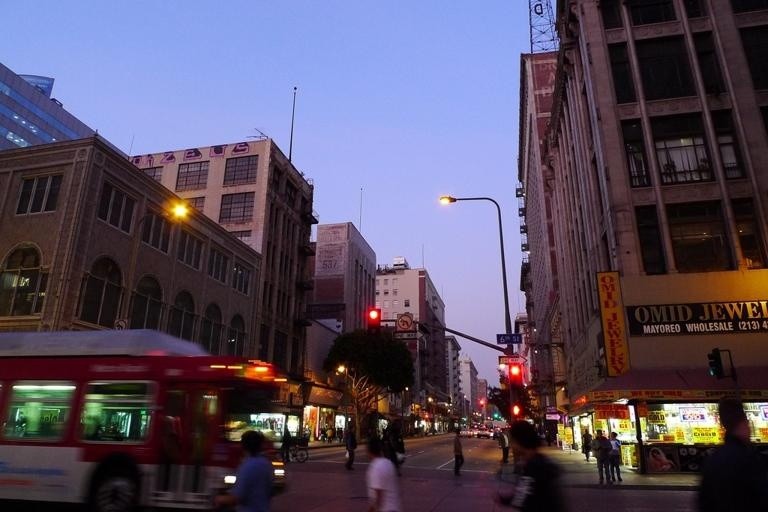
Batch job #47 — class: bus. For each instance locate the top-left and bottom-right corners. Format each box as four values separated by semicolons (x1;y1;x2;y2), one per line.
0;328;282;512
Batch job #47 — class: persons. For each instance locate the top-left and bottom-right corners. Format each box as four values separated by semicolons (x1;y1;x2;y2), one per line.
366;421;407;512
87;416;103;440
452;424;560;512
282;423;355;469
581;428;622;488
696;399;768;512
212;429;275;511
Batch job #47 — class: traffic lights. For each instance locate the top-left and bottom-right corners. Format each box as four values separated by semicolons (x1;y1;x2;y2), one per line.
367;306;383;333
507;362;523;388
705;345;724;382
479;397;486;408
509;400;523;417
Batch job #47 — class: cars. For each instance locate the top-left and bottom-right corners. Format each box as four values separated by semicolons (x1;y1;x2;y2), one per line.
458;416;511;441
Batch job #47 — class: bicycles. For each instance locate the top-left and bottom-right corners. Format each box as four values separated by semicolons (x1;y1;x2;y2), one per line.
275;440;309;463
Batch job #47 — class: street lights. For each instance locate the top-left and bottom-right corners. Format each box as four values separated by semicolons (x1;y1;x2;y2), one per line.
337;358;349;443
437;194;516;447
117;199;195;326
428;392;439;435
400;383;412;438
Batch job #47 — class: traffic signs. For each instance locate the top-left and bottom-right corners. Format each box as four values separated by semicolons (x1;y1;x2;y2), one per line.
498;352;527;367
496;333;523;345
392;329;425;340
